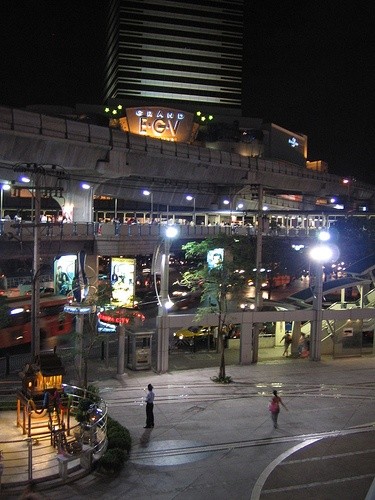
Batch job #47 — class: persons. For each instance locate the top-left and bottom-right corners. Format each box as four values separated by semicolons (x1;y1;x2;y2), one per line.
56;266;69;295
280;331;292;357
143;384;154;429
40;210;66;223
269;390;288;429
4;211;21;223
298;216;301;225
73;280;87;303
213;253;222;270
111;264;118;281
208;219;229;226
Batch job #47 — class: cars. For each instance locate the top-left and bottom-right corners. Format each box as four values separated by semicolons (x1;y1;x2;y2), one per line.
172;305;290;344
135;271;152;287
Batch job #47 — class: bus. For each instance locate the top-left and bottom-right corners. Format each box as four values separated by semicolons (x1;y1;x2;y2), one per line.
0;294;73;355
252;270;291;290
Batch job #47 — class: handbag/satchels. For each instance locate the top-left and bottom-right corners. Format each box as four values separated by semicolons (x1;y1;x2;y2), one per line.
268;403;278;411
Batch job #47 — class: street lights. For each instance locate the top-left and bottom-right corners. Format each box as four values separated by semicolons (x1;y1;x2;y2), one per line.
0;184;11;220
185;195;196;226
143;190;154;224
222;199;233;226
81;184;93;222
342;178;350;215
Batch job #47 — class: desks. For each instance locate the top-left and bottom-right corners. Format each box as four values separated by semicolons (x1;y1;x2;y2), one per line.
56;445;94;479
